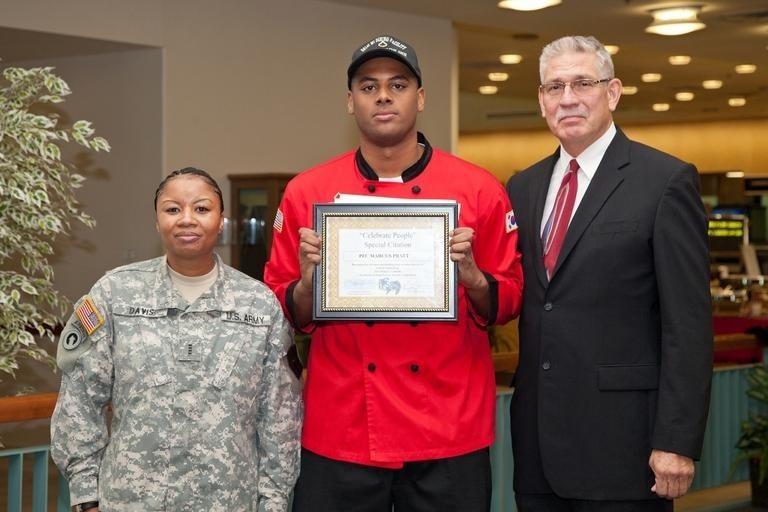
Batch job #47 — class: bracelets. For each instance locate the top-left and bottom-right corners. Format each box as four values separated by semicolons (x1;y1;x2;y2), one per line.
73;501;98;511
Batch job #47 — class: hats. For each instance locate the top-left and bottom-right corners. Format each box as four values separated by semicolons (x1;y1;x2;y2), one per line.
344;35;423;89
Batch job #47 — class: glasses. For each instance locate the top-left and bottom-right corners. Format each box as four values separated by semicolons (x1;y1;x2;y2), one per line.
541;75;612;98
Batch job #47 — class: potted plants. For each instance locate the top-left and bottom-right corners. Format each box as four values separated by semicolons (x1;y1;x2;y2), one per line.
725;364;768;508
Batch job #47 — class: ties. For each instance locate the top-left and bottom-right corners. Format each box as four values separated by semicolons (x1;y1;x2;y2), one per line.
539;155;580;283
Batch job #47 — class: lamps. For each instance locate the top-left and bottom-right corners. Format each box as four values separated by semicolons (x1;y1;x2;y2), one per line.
645;7;707;36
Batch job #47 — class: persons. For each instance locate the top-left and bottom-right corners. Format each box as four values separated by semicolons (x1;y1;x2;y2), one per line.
261;34;524;510
49;165;305;512
502;33;714;510
